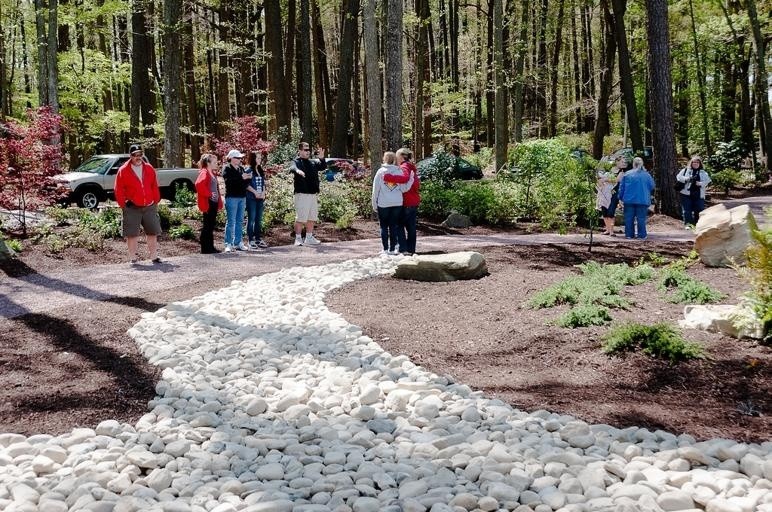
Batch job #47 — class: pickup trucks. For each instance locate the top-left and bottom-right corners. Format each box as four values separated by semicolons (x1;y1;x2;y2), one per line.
41;154;202;212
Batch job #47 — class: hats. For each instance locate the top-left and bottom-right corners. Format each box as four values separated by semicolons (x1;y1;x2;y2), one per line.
129;144;143;154
228;150;243;158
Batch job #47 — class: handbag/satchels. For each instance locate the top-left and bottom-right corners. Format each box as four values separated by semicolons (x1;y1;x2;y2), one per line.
673;179;686;192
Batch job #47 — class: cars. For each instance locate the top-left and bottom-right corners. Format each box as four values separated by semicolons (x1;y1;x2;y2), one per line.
311;157;371;180
412;156;487;181
498;145;654;179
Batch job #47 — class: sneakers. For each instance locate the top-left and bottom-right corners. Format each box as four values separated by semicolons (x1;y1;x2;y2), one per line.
249;241;258;249
255;240;267;248
233;242;248;251
295;238;304;246
224;243;231;252
305;237;321;245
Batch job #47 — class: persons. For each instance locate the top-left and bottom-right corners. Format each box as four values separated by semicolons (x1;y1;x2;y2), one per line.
289;142;327;246
222;150;252;253
372;150;415;254
245;151;269;248
676;157;712;231
381;148;421;256
194;153;223;253
596;156;627;236
114;145;169;264
617;157;656;239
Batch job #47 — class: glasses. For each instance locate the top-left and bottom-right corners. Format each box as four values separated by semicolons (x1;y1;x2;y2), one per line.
304;149;311;151
